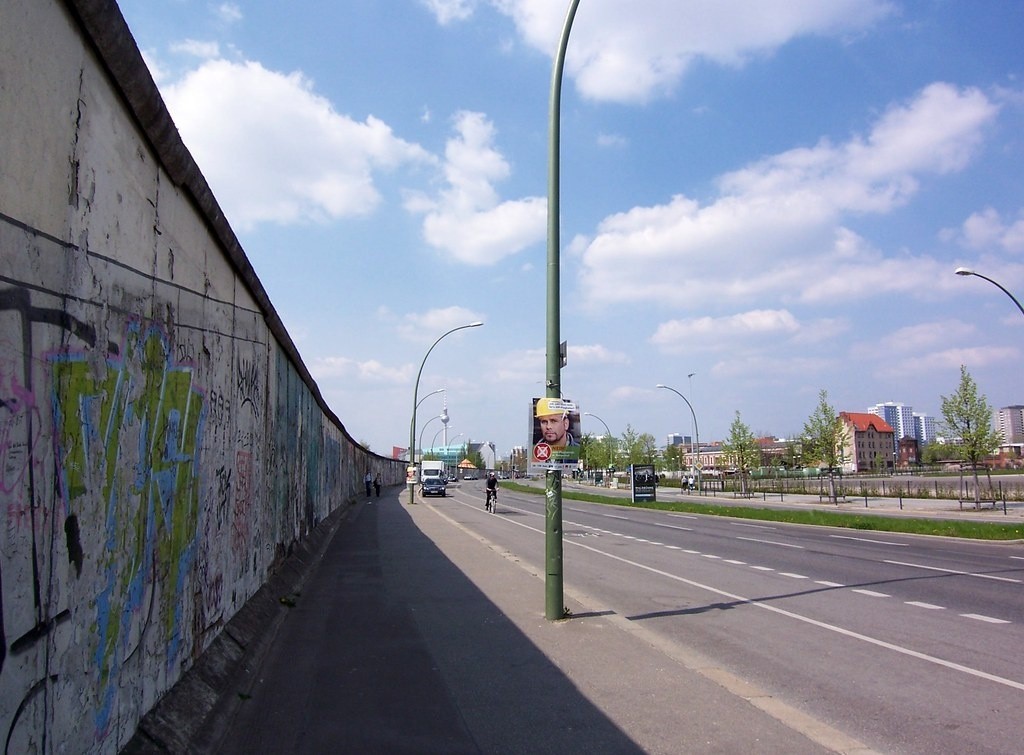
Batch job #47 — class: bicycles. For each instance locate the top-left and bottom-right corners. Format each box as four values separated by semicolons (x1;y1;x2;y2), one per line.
485;488;501;515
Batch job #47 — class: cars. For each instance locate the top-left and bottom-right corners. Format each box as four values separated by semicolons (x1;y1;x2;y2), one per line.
463;473;473;480
503;476;510;479
447;476;458;482
420;477;448;498
471;474;479;480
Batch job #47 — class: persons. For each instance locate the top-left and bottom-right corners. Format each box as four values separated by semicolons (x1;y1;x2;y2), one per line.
406;466;417;481
362;469;381;496
485;472;499;510
681;474;695;490
534;398;580;464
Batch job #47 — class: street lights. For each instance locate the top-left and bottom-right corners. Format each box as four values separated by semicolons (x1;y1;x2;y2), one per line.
409;389;444;491
688;371;695;480
446;433;464;483
656;383;702;498
431;426;454;460
418;414;450;476
409;321;485;505
584;412;613;478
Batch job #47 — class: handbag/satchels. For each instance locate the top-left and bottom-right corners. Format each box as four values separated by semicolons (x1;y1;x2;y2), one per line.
373;481;376;488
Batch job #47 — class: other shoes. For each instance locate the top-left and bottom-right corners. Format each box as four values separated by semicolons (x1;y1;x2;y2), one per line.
367;494;371;497
376;495;380;497
485;503;489;506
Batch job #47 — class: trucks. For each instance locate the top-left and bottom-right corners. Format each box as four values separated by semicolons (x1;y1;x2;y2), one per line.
419;460;446;487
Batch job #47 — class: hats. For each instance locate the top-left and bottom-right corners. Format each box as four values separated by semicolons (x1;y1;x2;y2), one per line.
490;472;495;475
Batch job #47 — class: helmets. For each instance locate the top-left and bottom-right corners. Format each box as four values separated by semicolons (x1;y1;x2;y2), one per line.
407;467;414;472
534;397;569;417
